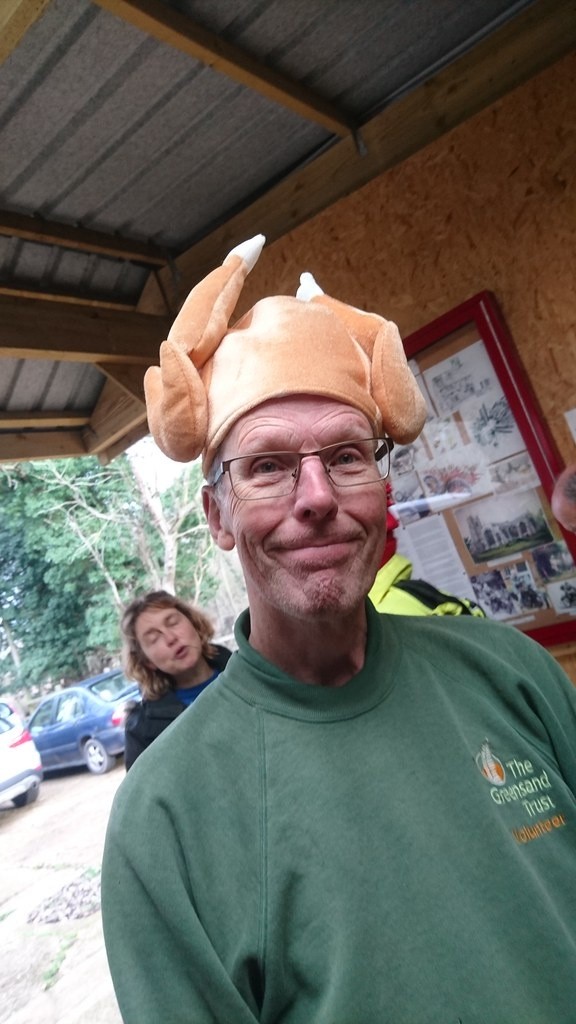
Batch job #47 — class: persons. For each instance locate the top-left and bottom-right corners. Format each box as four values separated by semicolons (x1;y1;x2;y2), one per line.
119;589;235;773
558;582;576;609
100;236;576;1024
534;546;558;581
426;464;474;495
509;571;543;609
367;479;487;619
551;464;576;534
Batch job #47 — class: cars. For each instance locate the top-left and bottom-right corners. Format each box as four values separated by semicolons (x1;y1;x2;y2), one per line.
0;697;44;808
27;667;141;774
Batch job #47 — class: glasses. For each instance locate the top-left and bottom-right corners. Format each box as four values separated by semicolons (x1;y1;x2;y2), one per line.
211;437;394;500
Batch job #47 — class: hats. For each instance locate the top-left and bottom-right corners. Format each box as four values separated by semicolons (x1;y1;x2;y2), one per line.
143;233;427;464
